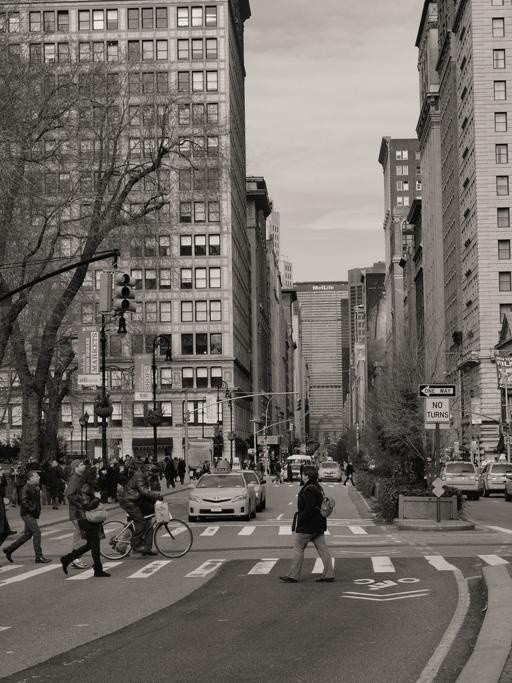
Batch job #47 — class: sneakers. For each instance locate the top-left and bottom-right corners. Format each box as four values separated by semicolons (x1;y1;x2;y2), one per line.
71;559;90;569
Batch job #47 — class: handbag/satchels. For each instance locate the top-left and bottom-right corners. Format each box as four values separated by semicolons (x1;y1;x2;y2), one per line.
85;503;106;523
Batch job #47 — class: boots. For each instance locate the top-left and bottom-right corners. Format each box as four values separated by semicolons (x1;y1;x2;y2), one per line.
60;549;81;574
92;563;111;577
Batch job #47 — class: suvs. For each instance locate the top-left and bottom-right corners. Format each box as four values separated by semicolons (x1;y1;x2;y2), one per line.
439;461;480;501
317;461;342;482
479;462;512;496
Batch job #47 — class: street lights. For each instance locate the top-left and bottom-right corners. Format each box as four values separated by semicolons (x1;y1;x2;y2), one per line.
84;411;89;458
147;334;172;462
69;425;74;455
214;379;231;469
96;307;127;467
79;414;84;457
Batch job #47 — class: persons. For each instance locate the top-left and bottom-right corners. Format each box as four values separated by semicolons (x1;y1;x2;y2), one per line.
192;455;307;486
0;456;187;578
279;466;337;583
342;458;376;487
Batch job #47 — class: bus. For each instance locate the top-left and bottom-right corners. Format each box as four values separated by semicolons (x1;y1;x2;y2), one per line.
286;454;315;464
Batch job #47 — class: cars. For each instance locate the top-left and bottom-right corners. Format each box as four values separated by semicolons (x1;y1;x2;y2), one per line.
188;471;258;522
233;470;268;511
283;463;305;480
504;471;512;500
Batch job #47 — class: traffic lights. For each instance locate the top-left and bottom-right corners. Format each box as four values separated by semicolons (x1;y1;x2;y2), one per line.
114;270;129;312
125;273;137;312
297;398;301;411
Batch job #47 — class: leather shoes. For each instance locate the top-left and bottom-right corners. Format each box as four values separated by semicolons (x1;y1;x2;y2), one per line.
279;576;297;583
35;558;52;563
142;551;157;556
316;578;334;582
3;547;13;563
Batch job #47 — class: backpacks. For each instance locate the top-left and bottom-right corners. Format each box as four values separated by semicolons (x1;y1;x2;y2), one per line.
303;485;335;517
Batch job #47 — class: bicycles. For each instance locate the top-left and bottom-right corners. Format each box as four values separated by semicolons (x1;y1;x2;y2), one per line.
98;492;194;559
